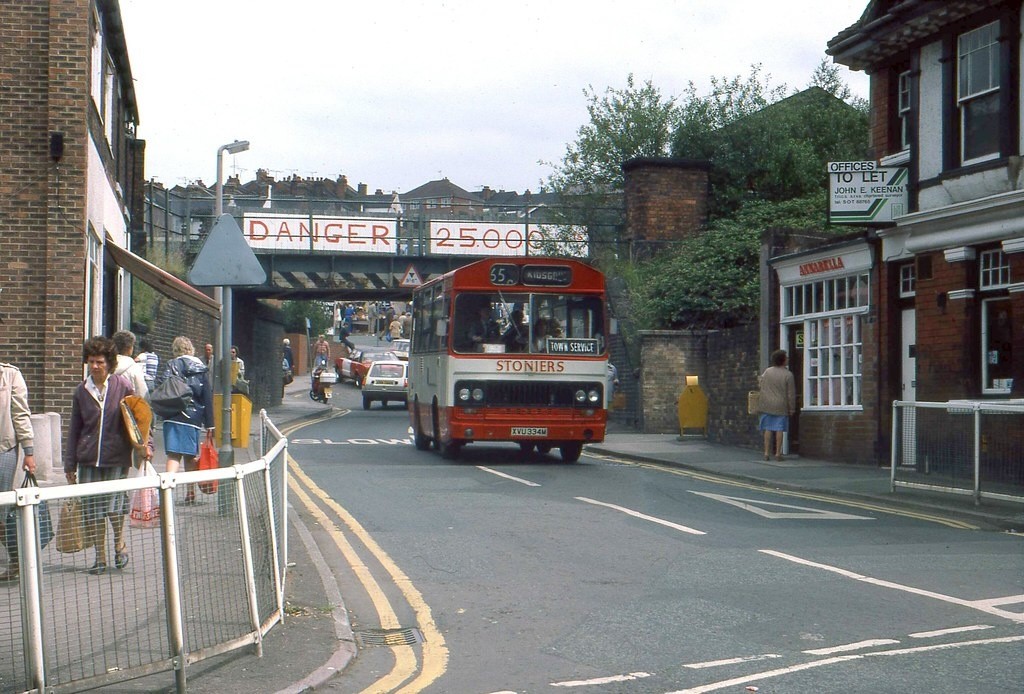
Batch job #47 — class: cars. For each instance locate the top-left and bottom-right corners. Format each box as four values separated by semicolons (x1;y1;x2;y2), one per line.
385;338;411;362
360;361;411;409
334;346;401;390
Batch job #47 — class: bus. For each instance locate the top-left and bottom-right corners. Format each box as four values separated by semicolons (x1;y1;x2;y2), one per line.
408;253;612;465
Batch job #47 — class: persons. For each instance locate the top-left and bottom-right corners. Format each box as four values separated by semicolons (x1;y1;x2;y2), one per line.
148;336;216;503
281;338;295;398
757;349;796;461
113;330;154;430
467;207;479;219
0;361;36;581
311;335;330;367
133;339;158;429
468;307;564;353
219;346;245;394
198;344;214;390
335;303;412;339
64;336;156;575
362;211;371;217
339;322;355;351
338;202;359;217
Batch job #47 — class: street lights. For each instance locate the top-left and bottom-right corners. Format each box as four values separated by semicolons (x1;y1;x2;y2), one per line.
216;138;252;518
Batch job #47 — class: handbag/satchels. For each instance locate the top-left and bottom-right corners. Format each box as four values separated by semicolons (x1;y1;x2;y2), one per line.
127;460;161;528
282;370;293;385
195;429;218;494
6;470;56;558
237;373;250;394
747;391;761;415
57;479;93;553
386;331;391;341
150;360;193;418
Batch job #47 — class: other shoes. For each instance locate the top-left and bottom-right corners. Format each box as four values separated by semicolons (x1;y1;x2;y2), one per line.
116;542;129;568
775;456;783;462
185;492;194;503
0;563;19;580
90;563;106;574
763;455;769;460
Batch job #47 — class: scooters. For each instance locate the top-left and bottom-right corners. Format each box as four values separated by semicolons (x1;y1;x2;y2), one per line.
307;359;339;405
